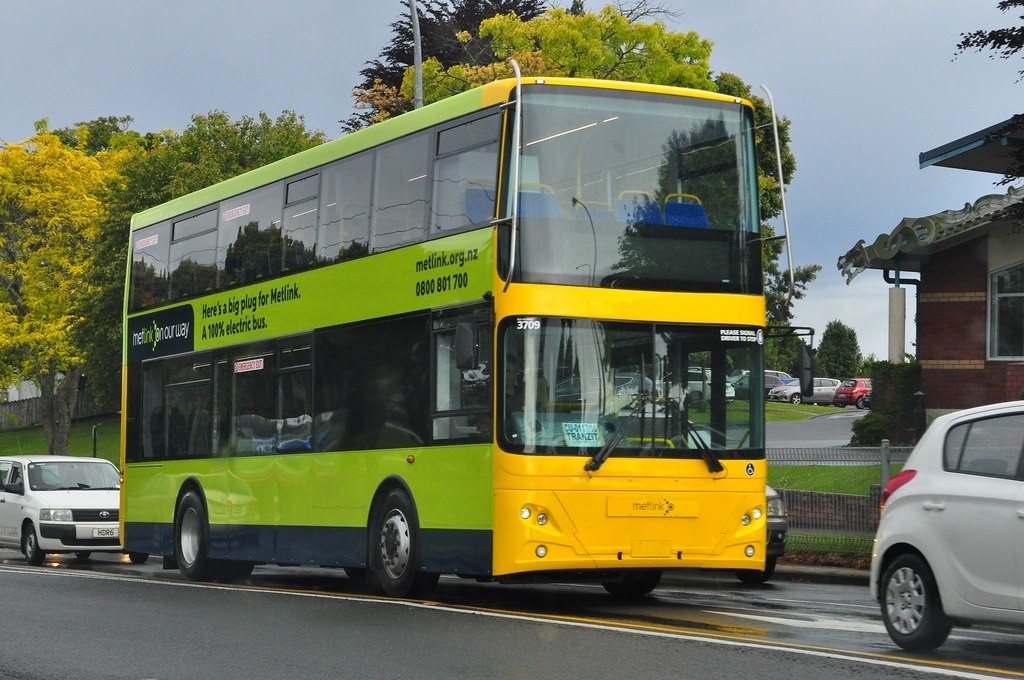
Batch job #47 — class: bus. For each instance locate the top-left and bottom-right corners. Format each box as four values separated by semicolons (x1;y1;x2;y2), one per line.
114;73;813;605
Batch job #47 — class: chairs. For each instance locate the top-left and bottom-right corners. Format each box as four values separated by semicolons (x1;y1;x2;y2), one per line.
463;177;711;229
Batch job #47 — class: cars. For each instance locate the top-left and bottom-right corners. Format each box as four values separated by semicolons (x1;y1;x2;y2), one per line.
730;374;784;400
832;377;874;408
554;372;661;408
625;483;790;593
769;377;842;405
868;399;1023;651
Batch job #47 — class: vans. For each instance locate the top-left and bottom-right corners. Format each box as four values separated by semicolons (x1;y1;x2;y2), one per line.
730;369;792;388
0;454;150;566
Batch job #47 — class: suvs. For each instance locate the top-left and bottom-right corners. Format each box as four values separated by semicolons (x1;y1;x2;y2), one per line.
657;365;735;407
